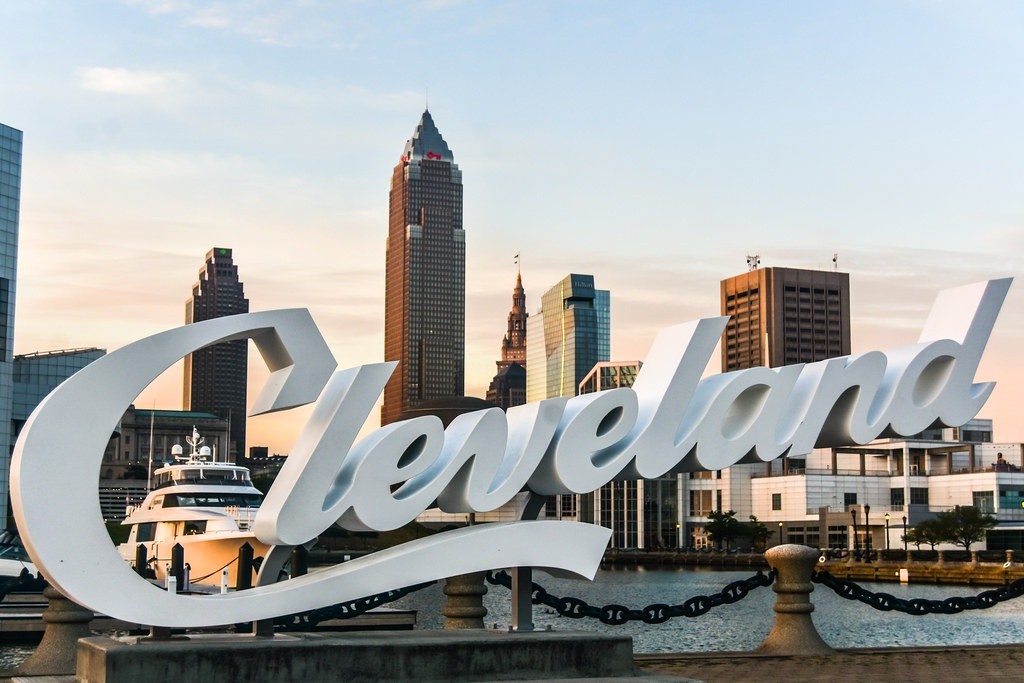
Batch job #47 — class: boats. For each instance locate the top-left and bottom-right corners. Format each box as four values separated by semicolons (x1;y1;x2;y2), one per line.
113;424;276;588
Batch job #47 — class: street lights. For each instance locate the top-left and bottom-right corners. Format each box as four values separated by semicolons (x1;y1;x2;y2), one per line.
778;520;783;546
884;513;892;557
676;522;681;553
851;508;860;563
864;502;872;565
902;514;908;555
